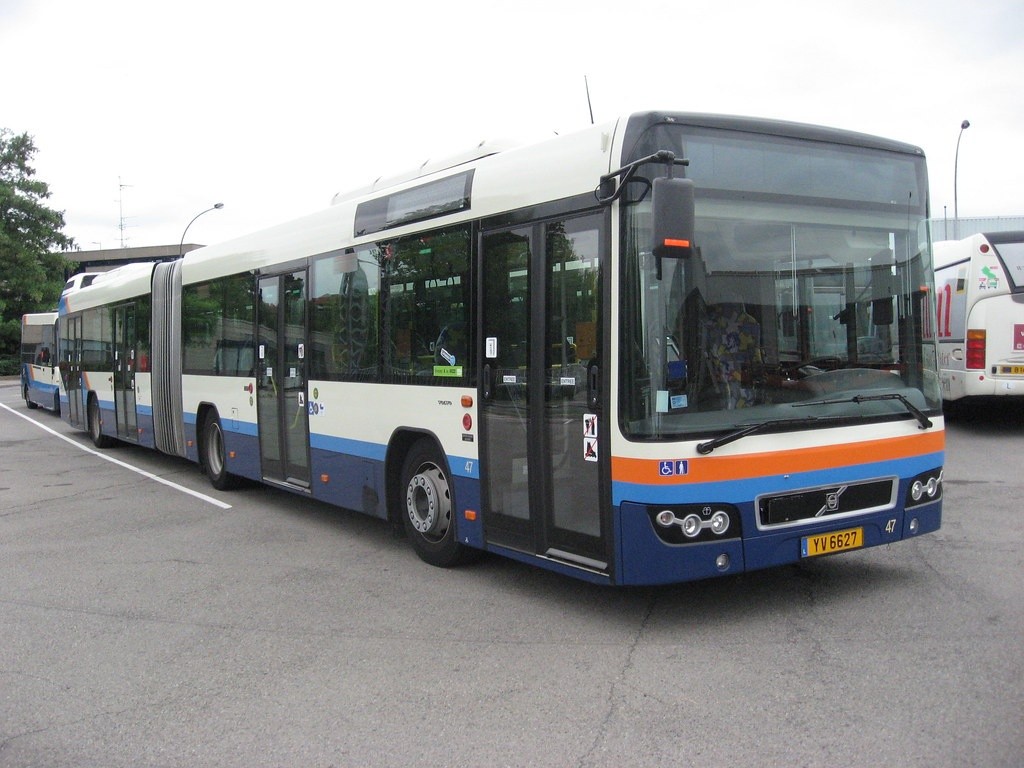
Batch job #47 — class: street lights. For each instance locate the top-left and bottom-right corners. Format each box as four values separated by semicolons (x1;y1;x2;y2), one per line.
954;118;971;218
178;201;224;257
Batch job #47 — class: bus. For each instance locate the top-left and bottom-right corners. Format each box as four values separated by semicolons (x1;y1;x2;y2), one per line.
58;107;951;587
921;229;1024;402
19;310;63;416
